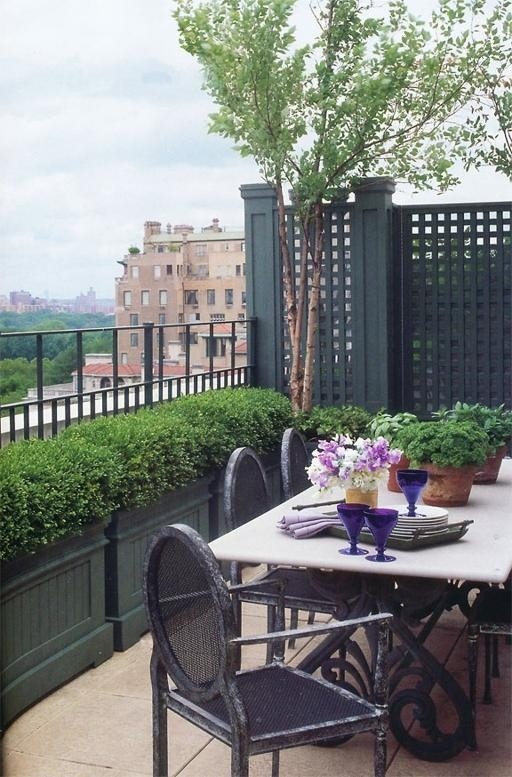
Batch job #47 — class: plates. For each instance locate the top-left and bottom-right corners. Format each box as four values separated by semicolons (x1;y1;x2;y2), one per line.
369;503;449;537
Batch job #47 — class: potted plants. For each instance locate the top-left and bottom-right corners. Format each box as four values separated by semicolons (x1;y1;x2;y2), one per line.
365;400;512;508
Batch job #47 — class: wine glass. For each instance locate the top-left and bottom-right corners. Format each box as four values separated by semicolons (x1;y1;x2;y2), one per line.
337;502;397;562
398;468;427;516
